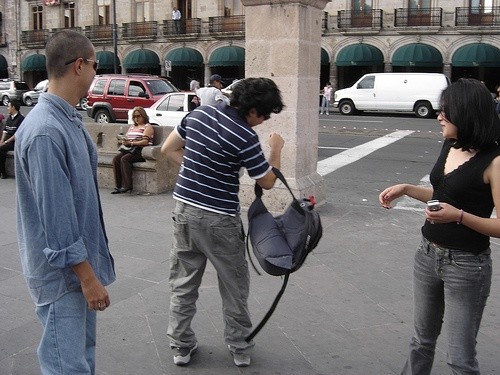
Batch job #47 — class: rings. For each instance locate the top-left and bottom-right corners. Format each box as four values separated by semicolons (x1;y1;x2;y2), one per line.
99;304;106;308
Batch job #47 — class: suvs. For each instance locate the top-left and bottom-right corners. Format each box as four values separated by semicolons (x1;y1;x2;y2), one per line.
86;73;179;124
0;78;31;106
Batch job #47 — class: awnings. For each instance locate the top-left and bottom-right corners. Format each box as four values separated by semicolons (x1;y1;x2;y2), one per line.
392;43;444;68
334;43;385;68
206;41;246;70
0;46;205;71
452;42;500;69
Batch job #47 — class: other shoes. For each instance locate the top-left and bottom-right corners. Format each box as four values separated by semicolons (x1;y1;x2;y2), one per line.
117;187;133;194
320;113;322;114
1;172;7;179
326;112;329;115
111;187;120;194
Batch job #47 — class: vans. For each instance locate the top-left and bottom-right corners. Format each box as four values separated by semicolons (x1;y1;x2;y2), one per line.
333;73;451;118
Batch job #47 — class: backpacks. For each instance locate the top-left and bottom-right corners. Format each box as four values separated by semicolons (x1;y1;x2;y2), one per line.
246;168;323;276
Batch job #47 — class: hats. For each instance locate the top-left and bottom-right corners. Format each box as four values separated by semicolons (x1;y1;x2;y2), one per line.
211;74;225;83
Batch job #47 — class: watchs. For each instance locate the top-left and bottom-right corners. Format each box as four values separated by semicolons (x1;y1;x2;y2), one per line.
4;140;8;144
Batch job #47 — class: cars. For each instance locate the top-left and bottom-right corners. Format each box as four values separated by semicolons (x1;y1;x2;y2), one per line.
221;79;244;94
128;93;201;127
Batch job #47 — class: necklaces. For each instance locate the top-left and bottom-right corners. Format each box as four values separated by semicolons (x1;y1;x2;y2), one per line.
187;77;200;91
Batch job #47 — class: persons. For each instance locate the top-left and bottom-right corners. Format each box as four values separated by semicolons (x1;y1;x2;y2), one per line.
159;76;284;367
172;6;182;35
377;77;500;374
0;102;25;179
319;82;333;115
109;106;156;195
14;29;117;374
481;79;500;113
196;73;226;109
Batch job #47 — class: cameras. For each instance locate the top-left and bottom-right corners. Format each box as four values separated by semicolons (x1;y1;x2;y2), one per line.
426;200;441;211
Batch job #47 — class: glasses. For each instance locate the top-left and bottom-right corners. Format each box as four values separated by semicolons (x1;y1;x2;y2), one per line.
263;115;270;120
65;57;99;72
134;114;141;118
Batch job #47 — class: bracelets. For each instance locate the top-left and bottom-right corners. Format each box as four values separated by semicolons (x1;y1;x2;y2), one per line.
456;209;464;225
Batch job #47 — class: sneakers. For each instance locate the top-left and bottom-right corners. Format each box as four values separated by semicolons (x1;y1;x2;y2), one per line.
173;341;197;365
227;344;251;366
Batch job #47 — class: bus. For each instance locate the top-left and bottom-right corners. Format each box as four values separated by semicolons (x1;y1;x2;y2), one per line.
22;79;88;111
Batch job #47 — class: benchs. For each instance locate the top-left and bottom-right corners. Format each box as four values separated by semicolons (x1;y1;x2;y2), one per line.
5;124;176;195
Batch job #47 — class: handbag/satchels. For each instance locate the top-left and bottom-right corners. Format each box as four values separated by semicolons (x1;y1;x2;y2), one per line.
119;145;139;153
